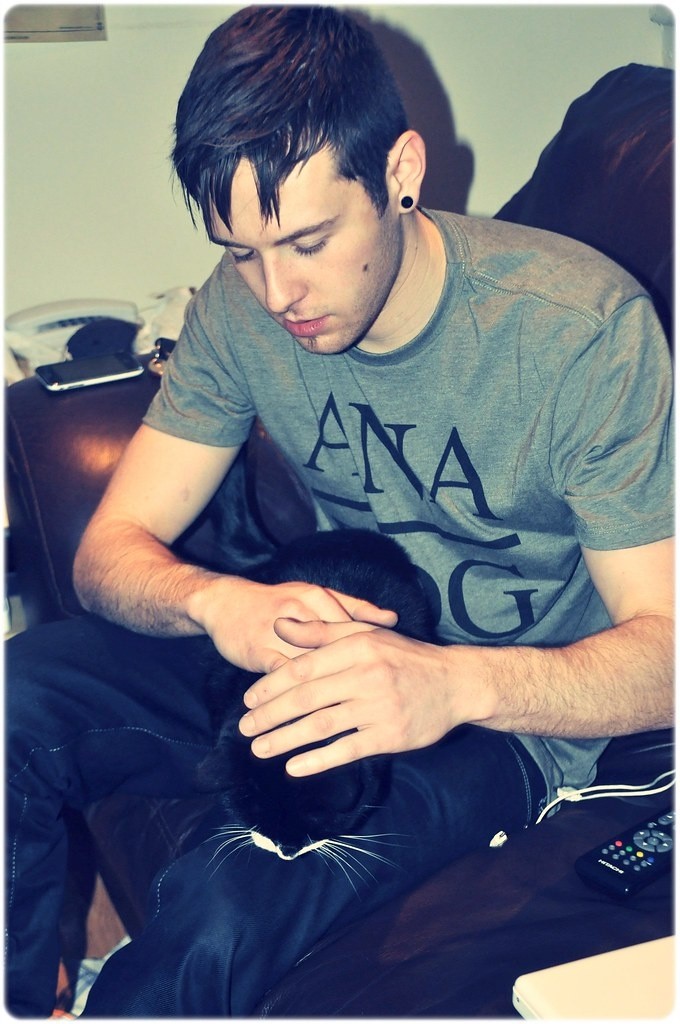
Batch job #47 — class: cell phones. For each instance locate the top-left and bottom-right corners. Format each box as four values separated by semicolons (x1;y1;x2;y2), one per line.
35;351;144;391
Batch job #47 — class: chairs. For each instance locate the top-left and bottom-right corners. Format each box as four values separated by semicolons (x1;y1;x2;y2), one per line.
5;66;675;1020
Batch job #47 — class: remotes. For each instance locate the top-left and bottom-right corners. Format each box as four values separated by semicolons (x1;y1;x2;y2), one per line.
573;809;676;901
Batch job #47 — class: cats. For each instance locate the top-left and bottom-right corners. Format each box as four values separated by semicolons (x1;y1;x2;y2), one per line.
209;528;438;903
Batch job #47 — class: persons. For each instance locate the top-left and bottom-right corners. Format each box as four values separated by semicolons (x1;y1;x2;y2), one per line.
5;5;676;1020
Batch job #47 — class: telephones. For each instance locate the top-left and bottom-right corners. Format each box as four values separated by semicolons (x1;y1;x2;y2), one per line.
4;298;144;348
